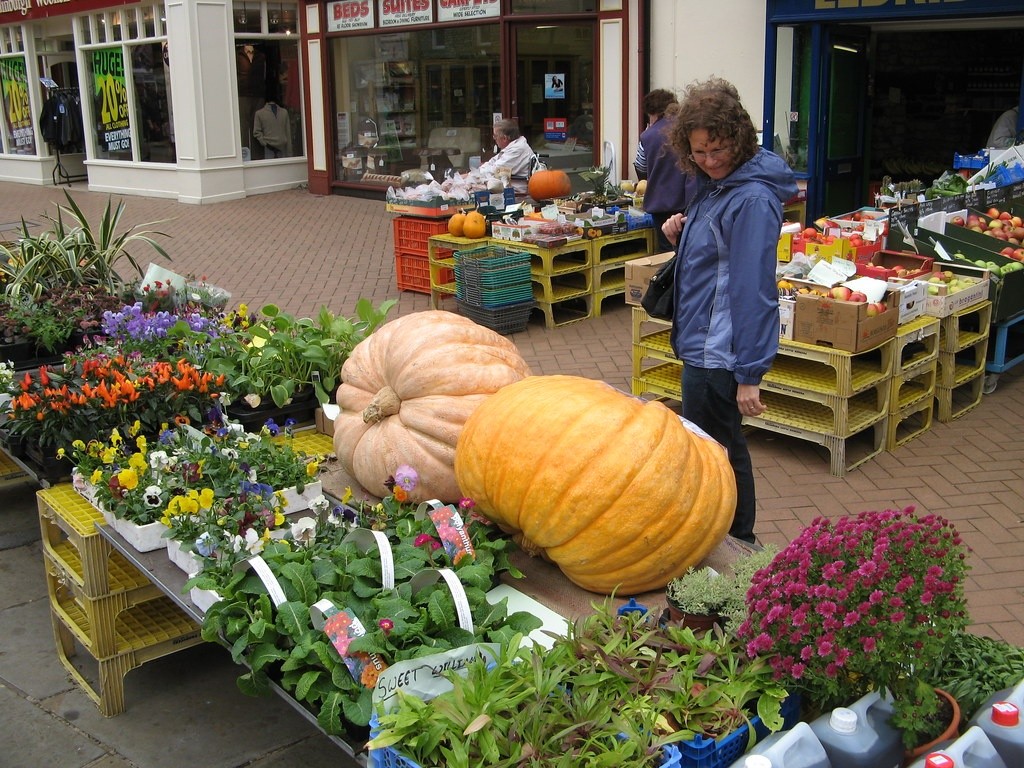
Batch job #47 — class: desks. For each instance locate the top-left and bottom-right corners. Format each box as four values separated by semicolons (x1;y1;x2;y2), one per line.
412;147;460;184
781;200;806;225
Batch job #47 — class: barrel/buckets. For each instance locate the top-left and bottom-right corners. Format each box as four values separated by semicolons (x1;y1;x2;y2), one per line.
808;686;906;768
964;678;1024;768
907;726;1006;768
727;721;832;768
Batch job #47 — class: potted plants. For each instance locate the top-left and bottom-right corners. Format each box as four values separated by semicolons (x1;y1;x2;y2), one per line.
166;298;400;433
352;542;811;768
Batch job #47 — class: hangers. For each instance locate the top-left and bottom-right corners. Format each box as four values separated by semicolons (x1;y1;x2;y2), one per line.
53;87;80;98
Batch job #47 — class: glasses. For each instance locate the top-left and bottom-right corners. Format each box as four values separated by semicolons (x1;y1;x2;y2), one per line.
685;148;735;159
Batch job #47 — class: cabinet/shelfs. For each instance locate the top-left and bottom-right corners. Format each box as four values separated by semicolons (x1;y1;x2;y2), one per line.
908;299;992;422
353;51;580;175
428;228;659;330
631;306;895;477
852;313;940;451
960;72;1021;111
36;432;334;717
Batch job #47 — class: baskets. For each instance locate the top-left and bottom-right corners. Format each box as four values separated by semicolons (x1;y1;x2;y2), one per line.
452;244;537;334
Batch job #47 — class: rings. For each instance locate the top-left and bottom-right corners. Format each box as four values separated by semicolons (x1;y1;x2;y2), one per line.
749;406;754;410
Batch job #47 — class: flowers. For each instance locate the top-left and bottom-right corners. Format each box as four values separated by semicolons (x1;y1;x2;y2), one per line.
736;504;974;750
0;187;295;452
57;415;544;738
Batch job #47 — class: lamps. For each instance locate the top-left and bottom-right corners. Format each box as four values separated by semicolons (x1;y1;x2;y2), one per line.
237;0;247;24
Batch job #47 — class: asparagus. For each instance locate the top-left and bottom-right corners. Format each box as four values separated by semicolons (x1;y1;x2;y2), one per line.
879;175;923;197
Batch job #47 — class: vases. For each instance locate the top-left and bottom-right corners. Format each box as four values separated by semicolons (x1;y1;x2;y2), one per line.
0;341;34;360
904;688;962;768
39;340;69;355
72;330;104;349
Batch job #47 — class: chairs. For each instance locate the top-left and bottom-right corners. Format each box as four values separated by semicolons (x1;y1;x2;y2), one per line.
401;126;482;182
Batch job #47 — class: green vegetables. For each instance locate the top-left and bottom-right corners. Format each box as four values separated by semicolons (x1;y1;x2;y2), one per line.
924;160;1010;201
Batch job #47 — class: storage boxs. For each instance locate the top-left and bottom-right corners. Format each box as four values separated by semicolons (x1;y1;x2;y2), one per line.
492;196;654;248
393;217;457;298
459;206;523;237
625;143;1024;353
386;188;516;217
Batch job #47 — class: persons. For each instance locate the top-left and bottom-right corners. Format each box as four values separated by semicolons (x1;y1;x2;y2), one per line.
253;102;293;159
460;119;535;194
661;73;799;544
633;88;697;254
238;46;262;95
986;105;1019;150
551;75;563;92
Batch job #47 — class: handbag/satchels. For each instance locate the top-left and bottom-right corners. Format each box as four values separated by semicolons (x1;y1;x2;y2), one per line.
640;242;676;322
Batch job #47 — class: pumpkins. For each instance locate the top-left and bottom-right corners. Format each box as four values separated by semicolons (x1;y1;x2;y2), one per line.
448;211;486;239
333;310;533;505
528;167;571;202
454;373;736;595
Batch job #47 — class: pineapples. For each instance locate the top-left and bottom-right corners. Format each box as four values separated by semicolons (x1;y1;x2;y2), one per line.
591;167;610;202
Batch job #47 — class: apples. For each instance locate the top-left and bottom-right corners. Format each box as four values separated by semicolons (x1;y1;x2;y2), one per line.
775;208;1024;296
777;280;886;317
